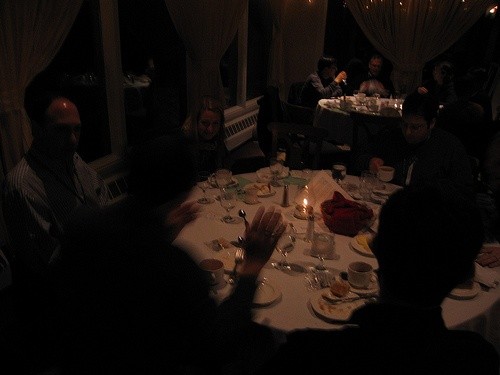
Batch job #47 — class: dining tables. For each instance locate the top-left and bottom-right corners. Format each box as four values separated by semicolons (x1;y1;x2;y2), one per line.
313;95;405;142
173;170;500;351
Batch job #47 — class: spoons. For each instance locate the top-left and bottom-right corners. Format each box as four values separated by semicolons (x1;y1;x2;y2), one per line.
322;294;377;304
238;235;244;245
239;209;250;226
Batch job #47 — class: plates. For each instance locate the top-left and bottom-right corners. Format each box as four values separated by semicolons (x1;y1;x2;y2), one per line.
255;164;312;184
223;270;282;304
337;182;401;202
243;182;275;196
350;234;377;257
208;177;239;188
334;266;380;296
309;287;366;322
447;281;481;300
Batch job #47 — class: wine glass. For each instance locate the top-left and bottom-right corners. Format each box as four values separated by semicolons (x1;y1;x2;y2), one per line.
220;187;237;224
343;76;347;85
272;220;297;271
307;228;336;287
194;170;213;204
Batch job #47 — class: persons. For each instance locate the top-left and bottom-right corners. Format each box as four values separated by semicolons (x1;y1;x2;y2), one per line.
3;94;112;260
479;104;500;191
346;53;396;116
85;201;287;375
434;60;492;181
332;94;473;187
160;98;228;207
418;61;457;124
276;180;500;375
295;55;347;122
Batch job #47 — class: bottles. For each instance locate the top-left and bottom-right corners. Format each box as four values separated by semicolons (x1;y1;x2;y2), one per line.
283;185;289;206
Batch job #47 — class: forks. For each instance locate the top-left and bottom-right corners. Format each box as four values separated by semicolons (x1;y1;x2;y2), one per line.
231;248;244;273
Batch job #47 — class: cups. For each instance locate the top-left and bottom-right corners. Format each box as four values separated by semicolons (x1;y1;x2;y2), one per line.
199;259;225;285
245;188;258;203
214;170;233;185
327;92;406;114
332;164;347;180
377;166;395;182
294;183;313;218
348;261;374;290
359;170;373;201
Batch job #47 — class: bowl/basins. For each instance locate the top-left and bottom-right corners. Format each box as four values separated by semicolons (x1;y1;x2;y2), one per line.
320;199;377;236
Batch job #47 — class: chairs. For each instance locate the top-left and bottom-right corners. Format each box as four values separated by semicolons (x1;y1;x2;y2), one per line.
269;81;484;163
